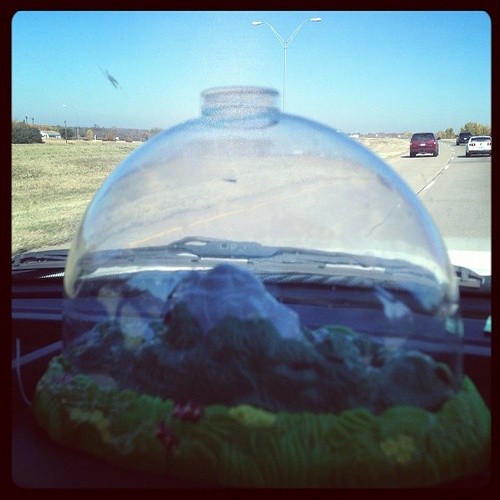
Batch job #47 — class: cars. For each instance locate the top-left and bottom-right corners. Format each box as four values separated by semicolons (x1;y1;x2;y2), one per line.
467;135;492;156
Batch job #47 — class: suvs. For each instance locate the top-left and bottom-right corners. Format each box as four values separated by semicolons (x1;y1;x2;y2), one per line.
409;133;440;158
456;133;471;145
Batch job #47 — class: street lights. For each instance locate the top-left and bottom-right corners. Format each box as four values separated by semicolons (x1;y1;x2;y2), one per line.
253;14;320;108
